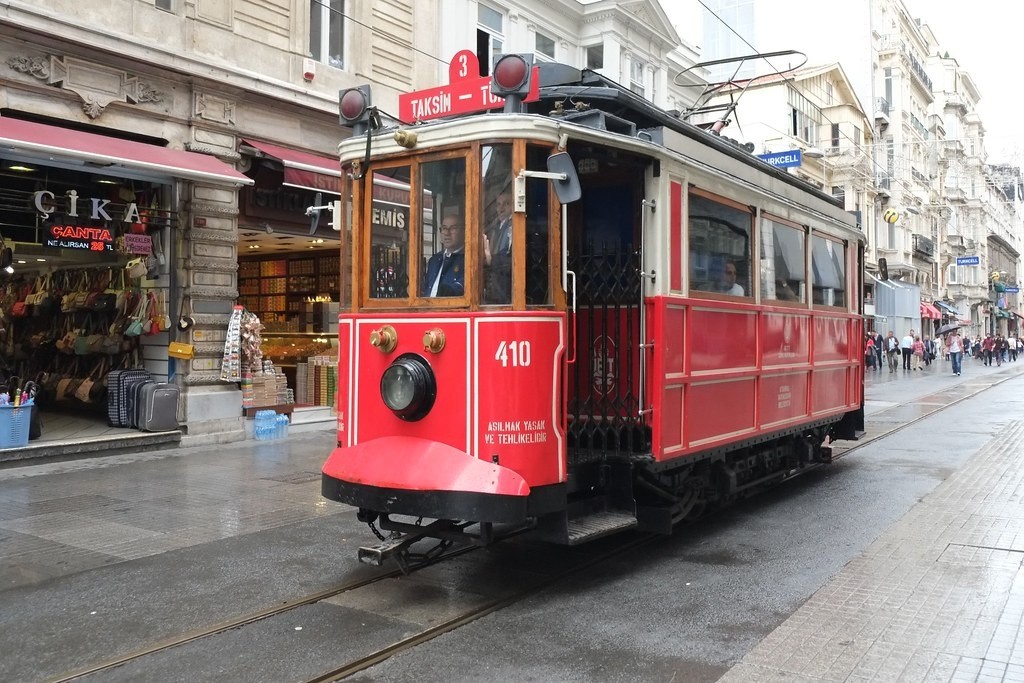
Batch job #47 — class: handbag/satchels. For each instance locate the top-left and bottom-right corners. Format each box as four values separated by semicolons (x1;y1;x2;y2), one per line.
866;347;876;367
0;179;195;407
897;349;901;355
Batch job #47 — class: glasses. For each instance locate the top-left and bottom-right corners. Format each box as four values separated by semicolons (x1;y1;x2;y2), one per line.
439;225;465;234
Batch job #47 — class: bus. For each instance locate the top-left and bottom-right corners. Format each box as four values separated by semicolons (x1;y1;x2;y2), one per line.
318;50;868;578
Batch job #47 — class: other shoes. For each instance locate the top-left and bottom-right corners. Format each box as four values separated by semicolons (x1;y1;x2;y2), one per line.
958;372;960;376
953;373;956;375
873;368;877;371
919;366;923;371
890;369;894;373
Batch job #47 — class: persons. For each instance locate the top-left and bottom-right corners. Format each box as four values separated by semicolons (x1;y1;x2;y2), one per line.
482;185;547;301
699;261;745;298
864;328;949;373
946;329;965;377
422;213;465;295
963;332;1024;366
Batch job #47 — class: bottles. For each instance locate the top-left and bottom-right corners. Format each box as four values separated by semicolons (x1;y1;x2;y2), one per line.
253;409;290;440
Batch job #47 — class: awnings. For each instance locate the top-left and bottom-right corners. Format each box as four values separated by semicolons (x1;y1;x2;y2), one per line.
1013;310;1024;320
935;300;963;315
0;116;254;187
920;302;941;319
241;138;435;213
998;309;1010;318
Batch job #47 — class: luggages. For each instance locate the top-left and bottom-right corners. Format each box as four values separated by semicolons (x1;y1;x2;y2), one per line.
108;369;180;432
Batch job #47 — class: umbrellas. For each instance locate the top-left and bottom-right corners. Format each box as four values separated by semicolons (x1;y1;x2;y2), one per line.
936;323;961;335
0;371;47;444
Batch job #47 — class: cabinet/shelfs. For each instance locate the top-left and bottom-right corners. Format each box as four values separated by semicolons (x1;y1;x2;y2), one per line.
238;248;340;321
